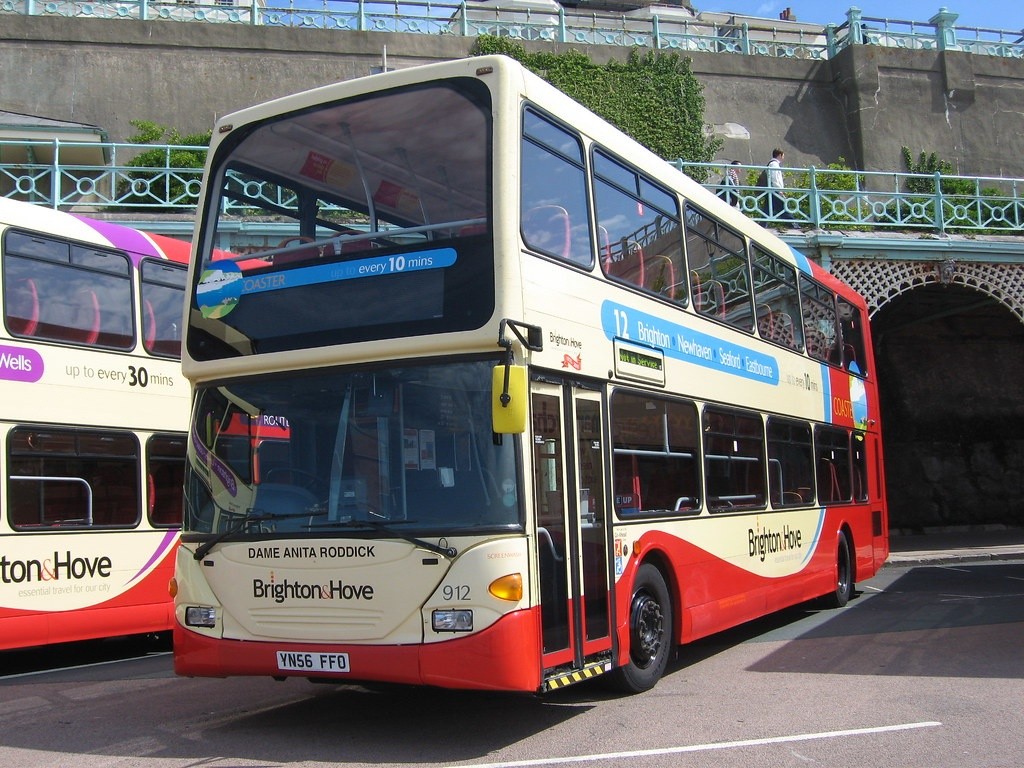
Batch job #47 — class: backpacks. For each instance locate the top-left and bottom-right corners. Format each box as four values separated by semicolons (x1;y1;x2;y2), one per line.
754;160;779;198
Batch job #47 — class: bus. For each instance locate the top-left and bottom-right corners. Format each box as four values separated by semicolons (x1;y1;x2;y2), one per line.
173;55;887;698
0;195;394;649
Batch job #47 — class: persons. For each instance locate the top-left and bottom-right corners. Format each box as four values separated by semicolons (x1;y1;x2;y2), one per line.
755;147;802;230
717;160;743;211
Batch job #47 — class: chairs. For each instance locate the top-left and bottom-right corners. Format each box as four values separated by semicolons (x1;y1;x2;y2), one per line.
3;274;181;524
256;199;862;523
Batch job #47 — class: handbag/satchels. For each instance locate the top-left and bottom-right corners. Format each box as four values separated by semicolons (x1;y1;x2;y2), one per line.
716;177;738;206
